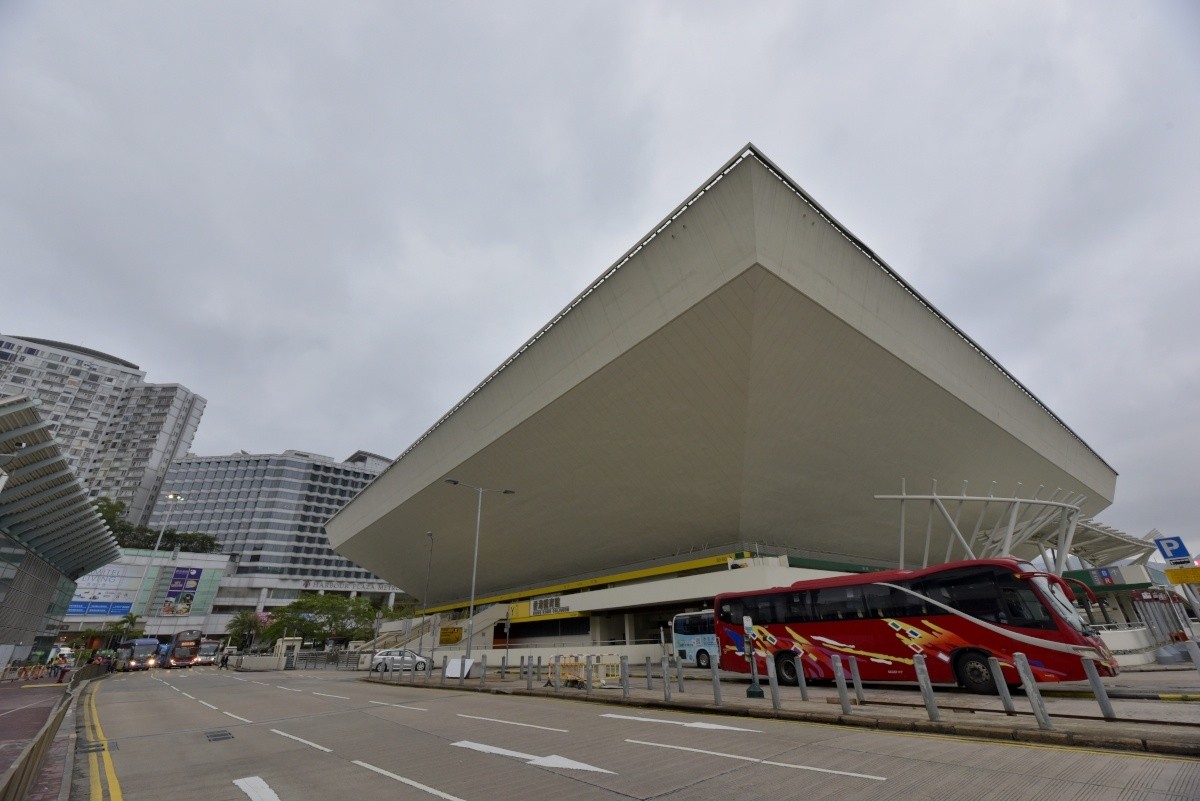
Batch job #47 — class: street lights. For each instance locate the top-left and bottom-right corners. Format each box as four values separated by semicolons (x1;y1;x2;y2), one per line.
130;492;184;619
417;530;434;653
443;478;516;659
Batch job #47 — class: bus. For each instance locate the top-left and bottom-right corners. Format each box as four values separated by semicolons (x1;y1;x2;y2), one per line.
115;639;161;672
194;639;221;667
671;608;719;669
167;629;203;668
713;554;1121;697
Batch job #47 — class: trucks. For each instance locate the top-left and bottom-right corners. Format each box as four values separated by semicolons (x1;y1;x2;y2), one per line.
156;643;172;667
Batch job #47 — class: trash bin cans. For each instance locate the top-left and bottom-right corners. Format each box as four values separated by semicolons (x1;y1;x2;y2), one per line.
284;655;294;670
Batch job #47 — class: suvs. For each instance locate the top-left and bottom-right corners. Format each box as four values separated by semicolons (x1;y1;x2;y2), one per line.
371;649;435;672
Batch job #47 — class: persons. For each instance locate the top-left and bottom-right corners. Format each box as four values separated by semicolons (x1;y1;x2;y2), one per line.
217;654;229;671
47;656;116;684
